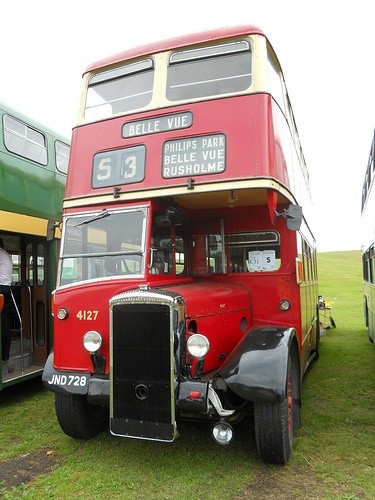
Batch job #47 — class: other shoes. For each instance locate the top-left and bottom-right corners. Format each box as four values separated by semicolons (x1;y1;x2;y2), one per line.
2;355;9;360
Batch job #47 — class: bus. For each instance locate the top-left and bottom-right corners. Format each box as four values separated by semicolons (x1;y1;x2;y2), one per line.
0;102;71;393
42;22;320;466
356;123;375;342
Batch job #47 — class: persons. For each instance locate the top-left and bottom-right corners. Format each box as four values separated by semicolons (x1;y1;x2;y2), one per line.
0;236;13;360
